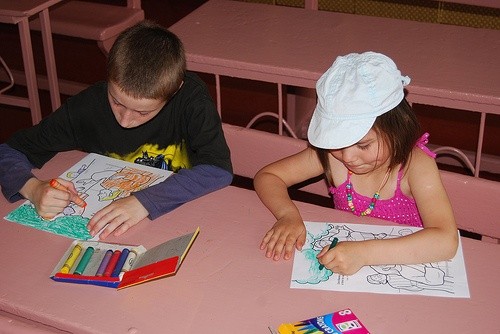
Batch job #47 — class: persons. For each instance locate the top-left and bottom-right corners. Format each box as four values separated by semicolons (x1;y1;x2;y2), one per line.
253;51;458;275
1;20;233;239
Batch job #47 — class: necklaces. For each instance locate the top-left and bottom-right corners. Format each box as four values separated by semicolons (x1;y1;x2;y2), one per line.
346;167;391;217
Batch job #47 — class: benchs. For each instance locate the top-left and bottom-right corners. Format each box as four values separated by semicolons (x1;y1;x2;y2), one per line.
0;0;500;244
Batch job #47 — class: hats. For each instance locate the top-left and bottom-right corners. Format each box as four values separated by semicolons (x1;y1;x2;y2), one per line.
307;51;410;149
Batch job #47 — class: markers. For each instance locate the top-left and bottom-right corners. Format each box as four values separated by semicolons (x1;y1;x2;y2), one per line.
73;246;94;275
60;243;83;274
319;237;338;270
96;248;137;278
50;179;87;208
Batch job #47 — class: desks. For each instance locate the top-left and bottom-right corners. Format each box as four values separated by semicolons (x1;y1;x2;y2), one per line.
0;0;63;125
0;153;500;334
168;0;500;175
